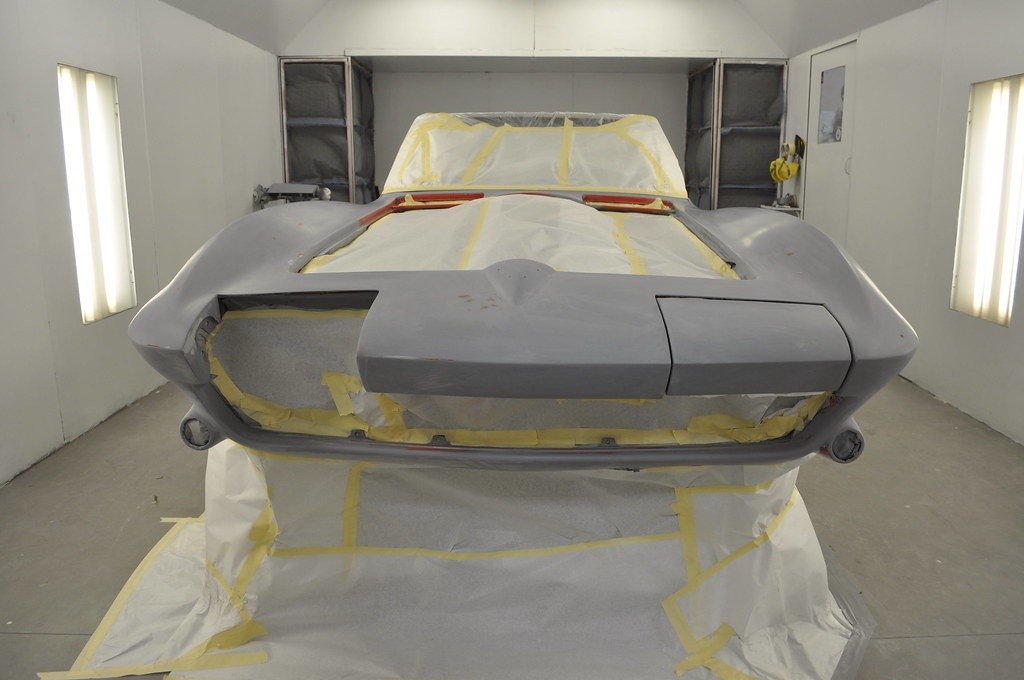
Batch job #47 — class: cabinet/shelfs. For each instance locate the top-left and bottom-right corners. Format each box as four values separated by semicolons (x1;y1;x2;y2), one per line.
280;57;377;203
685;59;789;212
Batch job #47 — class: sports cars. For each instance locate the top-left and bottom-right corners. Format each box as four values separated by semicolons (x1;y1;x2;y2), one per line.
127;114;920;466
